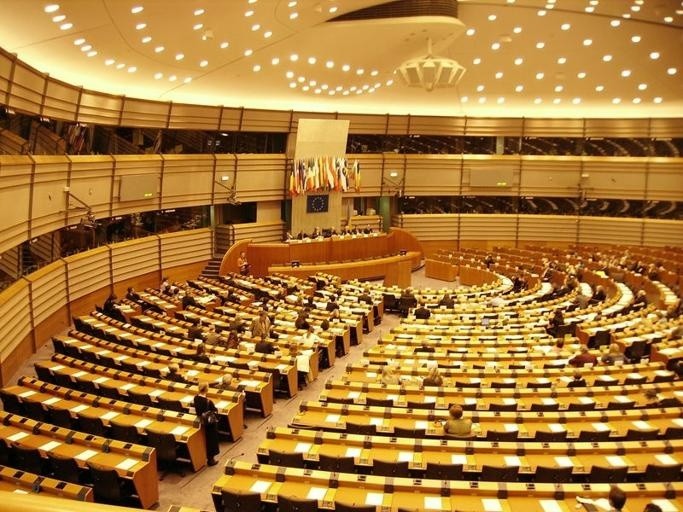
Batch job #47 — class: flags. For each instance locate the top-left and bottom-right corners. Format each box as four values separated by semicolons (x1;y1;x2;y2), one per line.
289;156;361;195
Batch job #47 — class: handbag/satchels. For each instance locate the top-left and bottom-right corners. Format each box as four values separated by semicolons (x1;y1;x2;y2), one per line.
201;410;219;425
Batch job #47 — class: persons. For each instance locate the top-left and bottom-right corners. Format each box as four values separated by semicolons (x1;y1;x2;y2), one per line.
103;251;371;362
400;252;658;336
284;223;373;241
608;483;663;512
194;381;219;466
566;369;586;387
422;367;443;385
165;363;198;386
216;374;237;391
602;342;632;366
444;404;470;434
571;346;599;368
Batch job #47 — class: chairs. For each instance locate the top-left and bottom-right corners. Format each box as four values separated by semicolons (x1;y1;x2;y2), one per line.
0;242;682;512
